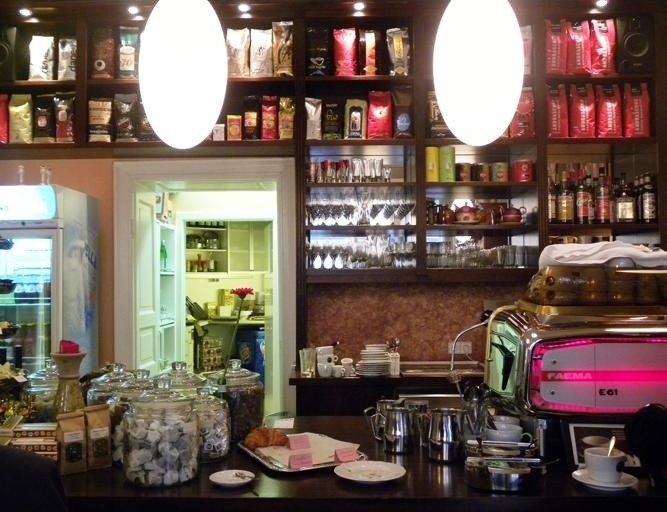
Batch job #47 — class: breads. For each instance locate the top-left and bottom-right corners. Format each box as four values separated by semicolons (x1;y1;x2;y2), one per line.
245;428;289;451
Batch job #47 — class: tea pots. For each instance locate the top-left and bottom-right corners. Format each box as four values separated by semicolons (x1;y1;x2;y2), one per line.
436;203;454;224
201;231;220;249
497;204;527;222
452;201;480;224
365;397;466;465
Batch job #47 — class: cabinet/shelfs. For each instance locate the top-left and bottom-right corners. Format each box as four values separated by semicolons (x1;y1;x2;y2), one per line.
184;219;228;272
159;224;177;366
0;0;295;158
295;11;666;281
228;219;272;273
0;236;54;380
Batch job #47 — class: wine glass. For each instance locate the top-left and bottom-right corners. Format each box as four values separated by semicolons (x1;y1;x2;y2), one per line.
306;186;416;226
306;231;416;270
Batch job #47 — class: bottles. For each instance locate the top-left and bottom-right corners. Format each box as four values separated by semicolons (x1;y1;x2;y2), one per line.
14;321;51;357
188;222;225;227
547;163;653;225
186;252;215;272
88;359;264;485
161;240;169;272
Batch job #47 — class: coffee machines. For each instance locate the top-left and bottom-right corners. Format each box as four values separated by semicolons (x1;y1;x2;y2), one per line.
448;306;666;470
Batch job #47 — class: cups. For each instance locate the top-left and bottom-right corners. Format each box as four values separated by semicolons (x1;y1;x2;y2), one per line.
315;346;355;376
425;237;530;267
297;349;314;374
583;449;627;483
522;261;666;304
305;157;392;183
549;235;614;245
482;412;533;444
581;436;610;452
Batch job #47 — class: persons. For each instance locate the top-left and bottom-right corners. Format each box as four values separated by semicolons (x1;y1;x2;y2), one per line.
0;446;67;512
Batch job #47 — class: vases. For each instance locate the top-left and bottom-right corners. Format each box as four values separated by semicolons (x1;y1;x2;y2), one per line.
49;350;87;421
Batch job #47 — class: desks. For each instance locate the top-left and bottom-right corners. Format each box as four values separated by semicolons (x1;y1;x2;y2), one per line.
188;318;263;368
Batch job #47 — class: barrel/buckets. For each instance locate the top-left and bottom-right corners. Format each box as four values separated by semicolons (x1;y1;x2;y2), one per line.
252;326;266;385
235;326;254;371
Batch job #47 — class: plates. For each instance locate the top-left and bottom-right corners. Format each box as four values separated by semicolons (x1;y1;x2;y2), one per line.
236;433;368;474
358;343;390;376
571;469;638;492
334;459;406;484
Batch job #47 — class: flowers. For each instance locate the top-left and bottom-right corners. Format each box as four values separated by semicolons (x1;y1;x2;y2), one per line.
218;287;254;385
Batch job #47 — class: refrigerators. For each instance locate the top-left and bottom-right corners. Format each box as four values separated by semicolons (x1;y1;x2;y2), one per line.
0;184;102;380
